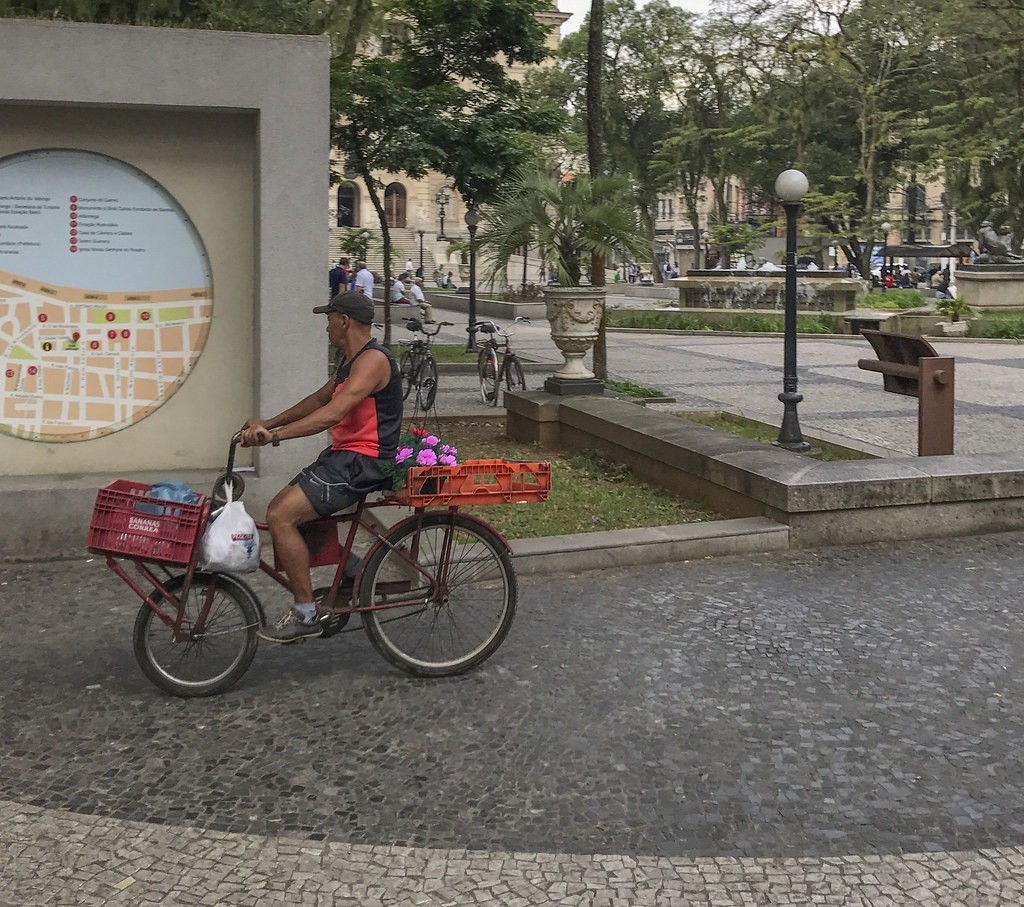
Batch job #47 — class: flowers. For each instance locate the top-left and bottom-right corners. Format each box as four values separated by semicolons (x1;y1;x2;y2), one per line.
380;427;457;492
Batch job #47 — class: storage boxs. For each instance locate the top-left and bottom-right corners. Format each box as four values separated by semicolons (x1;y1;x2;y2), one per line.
405;457;552;507
87;479;207;563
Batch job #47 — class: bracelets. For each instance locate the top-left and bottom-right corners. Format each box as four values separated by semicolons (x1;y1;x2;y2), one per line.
271;430;280;447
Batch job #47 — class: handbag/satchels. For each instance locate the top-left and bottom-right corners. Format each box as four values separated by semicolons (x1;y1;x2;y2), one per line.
200;482;260;574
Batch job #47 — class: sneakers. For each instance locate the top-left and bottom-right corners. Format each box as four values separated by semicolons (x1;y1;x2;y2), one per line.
256;607;323;643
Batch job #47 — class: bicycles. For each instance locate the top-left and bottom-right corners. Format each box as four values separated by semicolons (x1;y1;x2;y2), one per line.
86;428;520;700
332;323;383;374
397;317;455;412
465;316;530;407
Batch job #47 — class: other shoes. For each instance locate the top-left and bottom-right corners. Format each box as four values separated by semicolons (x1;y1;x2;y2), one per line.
424;320;436;324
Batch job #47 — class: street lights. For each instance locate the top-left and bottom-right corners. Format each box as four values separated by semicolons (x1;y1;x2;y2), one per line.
435;189;450;242
703;232;709;255
881;222;891;279
775;169;811;452
418;228;426;281
465;210;479;353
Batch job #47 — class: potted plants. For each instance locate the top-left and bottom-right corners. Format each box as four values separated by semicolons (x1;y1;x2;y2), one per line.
935;296;976;336
445;238;481;282
467;156;664;379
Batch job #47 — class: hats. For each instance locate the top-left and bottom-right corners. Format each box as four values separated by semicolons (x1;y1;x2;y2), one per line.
313;291;374;324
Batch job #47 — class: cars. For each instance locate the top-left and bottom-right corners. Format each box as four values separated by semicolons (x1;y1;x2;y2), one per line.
869;263;909;282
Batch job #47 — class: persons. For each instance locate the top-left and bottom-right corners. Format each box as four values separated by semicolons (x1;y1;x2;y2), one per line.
238;290;404;643
832;262;954;299
329;257;457;325
629;258;680;283
